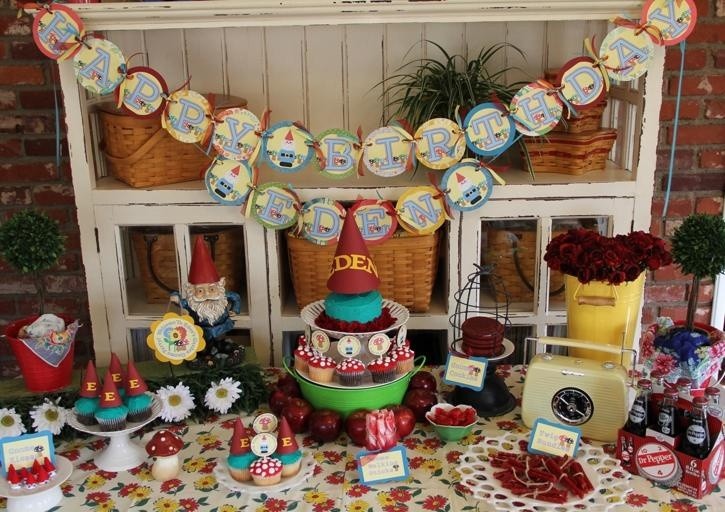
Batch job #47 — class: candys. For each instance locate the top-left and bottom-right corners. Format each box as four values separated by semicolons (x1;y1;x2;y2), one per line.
489;440;594;504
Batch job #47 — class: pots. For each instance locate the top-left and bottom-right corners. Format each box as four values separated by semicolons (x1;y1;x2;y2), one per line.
282;353;426;412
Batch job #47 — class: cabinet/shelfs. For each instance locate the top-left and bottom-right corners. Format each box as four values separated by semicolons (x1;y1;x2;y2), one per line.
50;0;677;373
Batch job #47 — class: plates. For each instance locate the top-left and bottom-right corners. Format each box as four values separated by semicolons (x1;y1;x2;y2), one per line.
211;455;316;493
302;299;410;335
294;341;411;389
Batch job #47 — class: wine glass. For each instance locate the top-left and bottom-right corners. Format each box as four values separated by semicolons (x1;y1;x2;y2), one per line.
67;394;163;472
0;454;72;512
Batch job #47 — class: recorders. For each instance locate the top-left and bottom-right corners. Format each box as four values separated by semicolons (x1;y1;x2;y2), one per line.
521;336;637;442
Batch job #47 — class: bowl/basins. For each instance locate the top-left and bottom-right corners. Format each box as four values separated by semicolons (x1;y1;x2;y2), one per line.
424;402;479;443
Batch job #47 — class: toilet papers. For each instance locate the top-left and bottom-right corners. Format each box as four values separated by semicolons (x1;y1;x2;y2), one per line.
710;270;725;332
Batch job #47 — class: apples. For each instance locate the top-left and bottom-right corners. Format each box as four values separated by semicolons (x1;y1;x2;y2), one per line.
271;376;342;442
346;371;438;444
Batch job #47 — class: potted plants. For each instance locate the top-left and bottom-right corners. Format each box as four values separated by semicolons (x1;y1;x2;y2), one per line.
643;210;725;397
0;205;80;393
361;35;534;161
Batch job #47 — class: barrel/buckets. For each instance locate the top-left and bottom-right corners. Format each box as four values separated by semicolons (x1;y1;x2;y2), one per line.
1;312;84;391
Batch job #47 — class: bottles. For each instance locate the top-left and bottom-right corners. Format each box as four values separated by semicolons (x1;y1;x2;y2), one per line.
657;387;681;437
704;385;724;419
620;435;635;468
684;397;711;457
650;371;664;395
676;377;693;403
625;378;654;434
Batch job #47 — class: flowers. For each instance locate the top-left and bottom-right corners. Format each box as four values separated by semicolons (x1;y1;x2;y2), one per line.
538;221;672;285
0;362;270;445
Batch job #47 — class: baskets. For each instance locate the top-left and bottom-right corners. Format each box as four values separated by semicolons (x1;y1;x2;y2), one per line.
482;229;598;303
286;230;441;313
93;93;248;189
131;228;243;305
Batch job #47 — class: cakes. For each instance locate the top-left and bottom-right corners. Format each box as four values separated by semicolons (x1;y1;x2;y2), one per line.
7;457;56;490
294;335;414;386
227;449;303;485
325;291;385;323
73;387;153;431
462;317;506;354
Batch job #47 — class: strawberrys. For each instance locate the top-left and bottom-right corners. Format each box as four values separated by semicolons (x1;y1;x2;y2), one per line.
427;404;475;427
